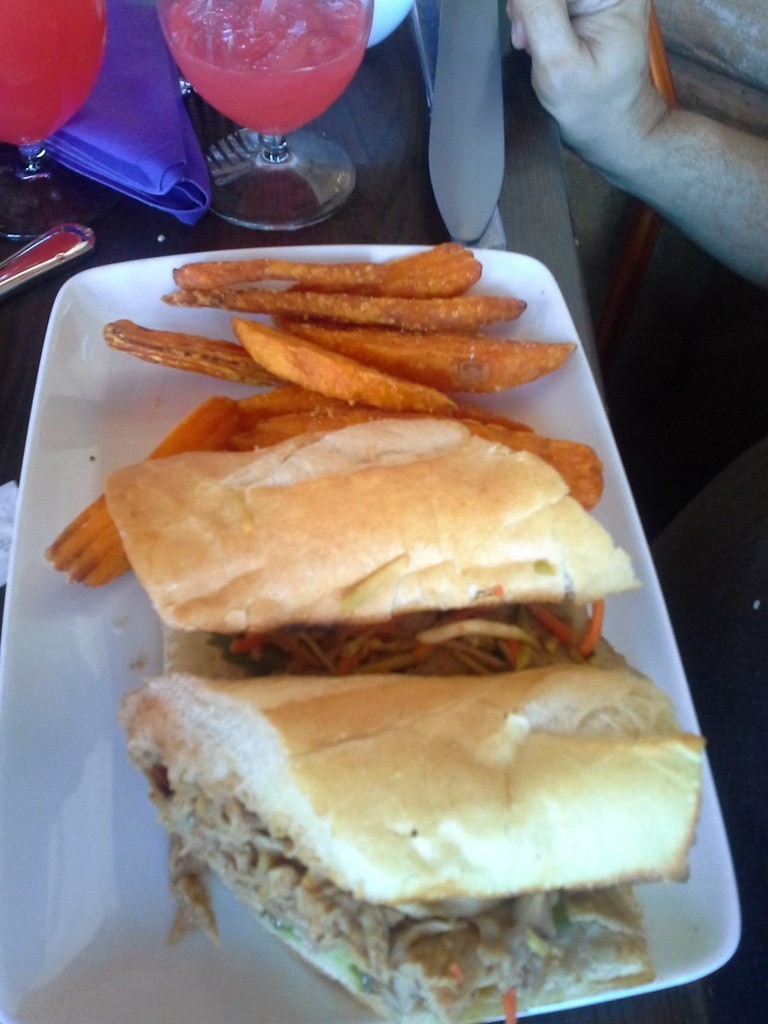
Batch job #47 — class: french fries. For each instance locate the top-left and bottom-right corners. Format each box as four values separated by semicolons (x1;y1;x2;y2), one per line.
47;244;606;587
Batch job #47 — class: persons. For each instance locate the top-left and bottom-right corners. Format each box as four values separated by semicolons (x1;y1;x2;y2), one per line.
505;0;767;282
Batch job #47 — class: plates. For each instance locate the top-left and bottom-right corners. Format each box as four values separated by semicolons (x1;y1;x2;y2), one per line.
0;247;742;1024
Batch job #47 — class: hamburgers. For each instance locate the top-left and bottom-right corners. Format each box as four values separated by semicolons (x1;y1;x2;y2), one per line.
102;417;706;1024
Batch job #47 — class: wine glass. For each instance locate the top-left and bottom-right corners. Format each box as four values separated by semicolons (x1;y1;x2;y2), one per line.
157;0;376;232
0;0;120;242
